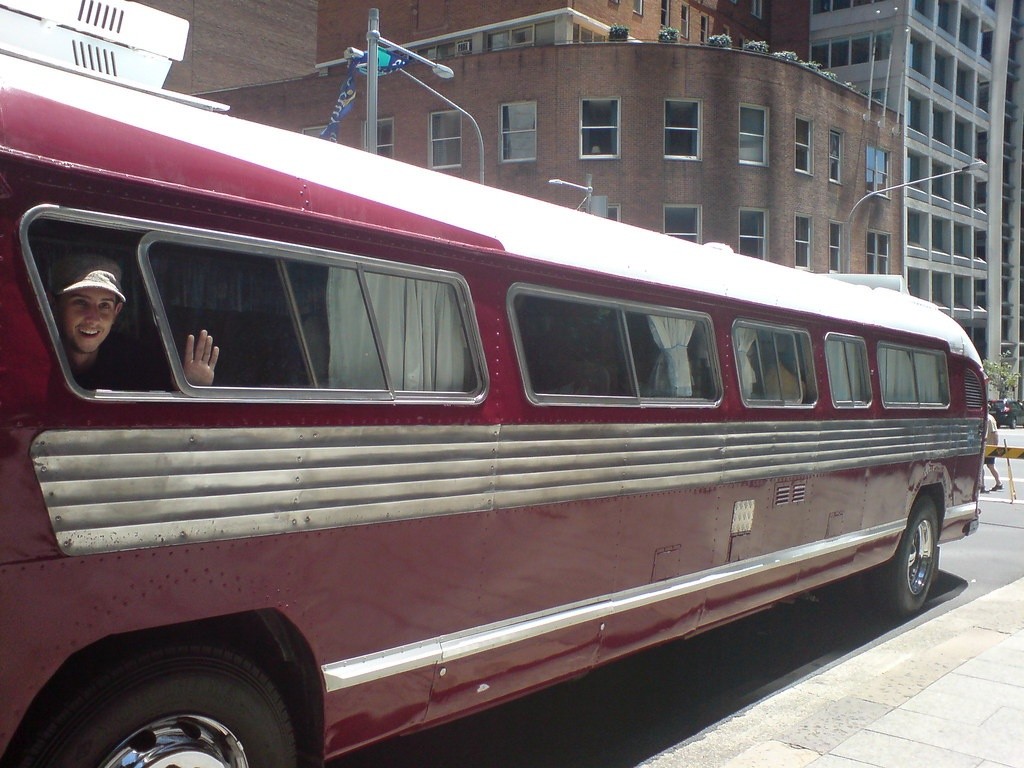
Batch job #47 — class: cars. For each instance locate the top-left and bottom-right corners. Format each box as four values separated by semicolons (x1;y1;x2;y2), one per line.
987;398;1024;429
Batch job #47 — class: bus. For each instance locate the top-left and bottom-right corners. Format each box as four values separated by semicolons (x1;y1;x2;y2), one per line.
1;1;988;768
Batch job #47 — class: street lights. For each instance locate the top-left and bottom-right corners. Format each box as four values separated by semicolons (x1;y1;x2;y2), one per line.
343;47;486;185
548;174;593;214
846;160;985;274
362;6;458;158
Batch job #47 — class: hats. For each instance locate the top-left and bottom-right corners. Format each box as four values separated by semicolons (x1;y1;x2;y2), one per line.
988;404;991;409
52;254;127;304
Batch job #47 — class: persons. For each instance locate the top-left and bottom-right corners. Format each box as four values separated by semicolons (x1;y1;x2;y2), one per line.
51;254;219;390
981;403;1003;493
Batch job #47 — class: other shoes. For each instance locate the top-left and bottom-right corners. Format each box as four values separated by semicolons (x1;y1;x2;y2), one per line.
992;485;1003;491
981;487;990;493
